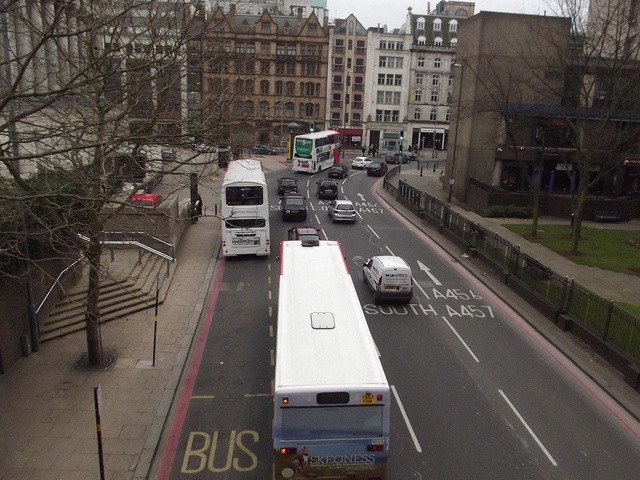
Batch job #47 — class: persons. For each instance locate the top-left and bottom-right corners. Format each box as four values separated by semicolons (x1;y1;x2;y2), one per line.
194;193;204;217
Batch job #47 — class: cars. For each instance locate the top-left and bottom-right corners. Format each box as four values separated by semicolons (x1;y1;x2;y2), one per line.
328;165;349;178
251;144;279;155
196;144;215;152
287;227;320;240
328;200;356;224
277;178;298;195
281;193;307;221
352;156;373;170
367;161;387;176
316;178;338;198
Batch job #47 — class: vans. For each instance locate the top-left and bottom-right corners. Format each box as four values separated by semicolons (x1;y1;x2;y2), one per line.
385;152;409;164
361;255;414;306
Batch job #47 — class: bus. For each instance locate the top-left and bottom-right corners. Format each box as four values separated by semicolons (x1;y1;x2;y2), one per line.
271;240;392;480
291;129;342;173
220;158;270;259
